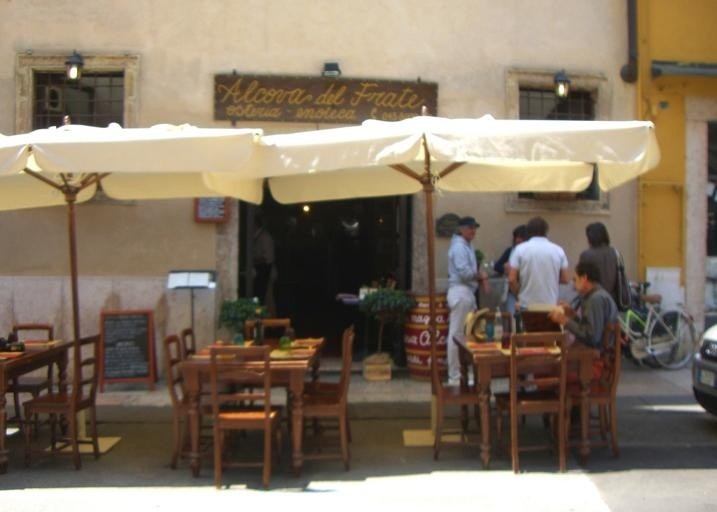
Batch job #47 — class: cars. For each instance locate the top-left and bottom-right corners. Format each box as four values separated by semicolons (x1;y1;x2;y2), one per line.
691;323;717;417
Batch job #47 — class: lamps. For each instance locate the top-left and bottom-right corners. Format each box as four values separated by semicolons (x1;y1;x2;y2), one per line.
63;50;84;85
553;69;571;102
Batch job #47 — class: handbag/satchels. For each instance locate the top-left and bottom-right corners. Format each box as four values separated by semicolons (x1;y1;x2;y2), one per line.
614;249;634;313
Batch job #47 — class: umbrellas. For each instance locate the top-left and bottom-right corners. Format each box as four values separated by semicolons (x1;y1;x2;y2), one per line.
0;116;265;337
252;106;663;436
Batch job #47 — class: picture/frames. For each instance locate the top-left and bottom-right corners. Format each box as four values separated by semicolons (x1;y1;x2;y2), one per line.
193;197;232;224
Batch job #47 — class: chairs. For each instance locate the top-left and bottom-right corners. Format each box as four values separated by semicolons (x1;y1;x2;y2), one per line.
181;327;248;439
427;326;492;460
7;323;55;433
513;309;548;333
303;332;355;472
286;323;355;442
551;321;621;458
22;333;101;471
244;318;292;406
162;334;238;470
492;329;570;474
460;309;527;427
208;344;281;488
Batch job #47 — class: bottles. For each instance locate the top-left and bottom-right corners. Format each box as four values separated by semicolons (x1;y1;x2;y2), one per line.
253;309;264;346
494;307;504;342
512;303;522;348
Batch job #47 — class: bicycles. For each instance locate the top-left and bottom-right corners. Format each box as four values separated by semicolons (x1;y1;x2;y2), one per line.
618;293;700;371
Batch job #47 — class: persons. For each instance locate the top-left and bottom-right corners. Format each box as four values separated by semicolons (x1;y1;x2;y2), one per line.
547;262;619;385
445;215;490;386
493;224;528;330
245;213;278;314
507;215;571;331
579;221;626;311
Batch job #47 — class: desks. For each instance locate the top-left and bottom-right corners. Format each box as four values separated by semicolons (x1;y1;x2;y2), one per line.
0;336;75;475
176;336;326;477
452;333;601;468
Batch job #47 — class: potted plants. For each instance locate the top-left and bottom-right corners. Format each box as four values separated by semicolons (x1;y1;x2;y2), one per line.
358;288;417;381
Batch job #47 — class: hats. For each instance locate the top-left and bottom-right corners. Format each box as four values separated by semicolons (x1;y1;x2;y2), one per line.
457;214;481;230
